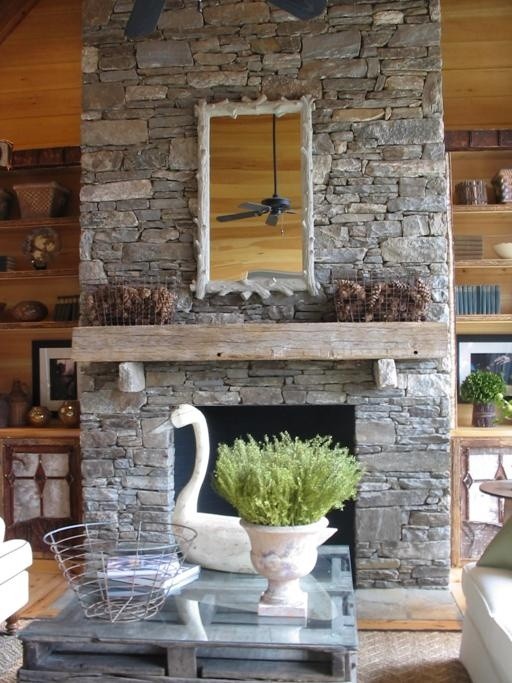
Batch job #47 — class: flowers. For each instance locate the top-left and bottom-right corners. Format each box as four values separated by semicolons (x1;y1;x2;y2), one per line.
20;228;60;262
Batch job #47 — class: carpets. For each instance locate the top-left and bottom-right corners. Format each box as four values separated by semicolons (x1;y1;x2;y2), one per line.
0;617;470;683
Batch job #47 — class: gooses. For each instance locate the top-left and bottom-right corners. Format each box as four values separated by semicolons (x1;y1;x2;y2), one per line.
147;403;339;578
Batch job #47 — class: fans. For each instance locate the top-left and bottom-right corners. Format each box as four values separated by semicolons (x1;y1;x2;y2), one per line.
125;0;329;39
216;114;300;235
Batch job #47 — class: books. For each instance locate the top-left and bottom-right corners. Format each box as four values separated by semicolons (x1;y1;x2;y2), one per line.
455;284;501;315
95;552;201;598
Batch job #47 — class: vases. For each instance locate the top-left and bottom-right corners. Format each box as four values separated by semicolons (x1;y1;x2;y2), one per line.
33;260;48;270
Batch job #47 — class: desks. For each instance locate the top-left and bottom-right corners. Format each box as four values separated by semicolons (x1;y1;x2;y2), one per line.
479;480;512;518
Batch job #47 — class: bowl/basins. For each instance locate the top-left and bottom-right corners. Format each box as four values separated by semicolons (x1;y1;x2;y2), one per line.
493;243;512;259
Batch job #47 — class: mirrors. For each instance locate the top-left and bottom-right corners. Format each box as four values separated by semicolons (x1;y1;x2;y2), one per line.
189;94;320;300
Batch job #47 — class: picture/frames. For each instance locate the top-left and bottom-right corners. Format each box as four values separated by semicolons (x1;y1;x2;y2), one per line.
32;339;81;417
456;334;512;403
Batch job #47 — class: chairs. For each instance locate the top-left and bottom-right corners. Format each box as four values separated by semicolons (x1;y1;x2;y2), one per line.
457;515;512;683
0;516;33;635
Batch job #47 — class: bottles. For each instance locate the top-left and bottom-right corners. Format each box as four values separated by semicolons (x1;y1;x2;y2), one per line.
9;380;80;426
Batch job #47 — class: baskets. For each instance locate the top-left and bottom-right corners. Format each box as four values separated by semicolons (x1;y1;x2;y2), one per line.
331;273;433;323
44;523;197;624
79;275;179;325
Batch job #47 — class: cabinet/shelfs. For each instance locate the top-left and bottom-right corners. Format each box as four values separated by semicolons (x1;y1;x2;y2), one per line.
446;150;512;567
0;164;82;556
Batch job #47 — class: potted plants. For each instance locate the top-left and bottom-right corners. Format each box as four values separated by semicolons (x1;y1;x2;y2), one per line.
212;430;363;618
460;372;507;427
